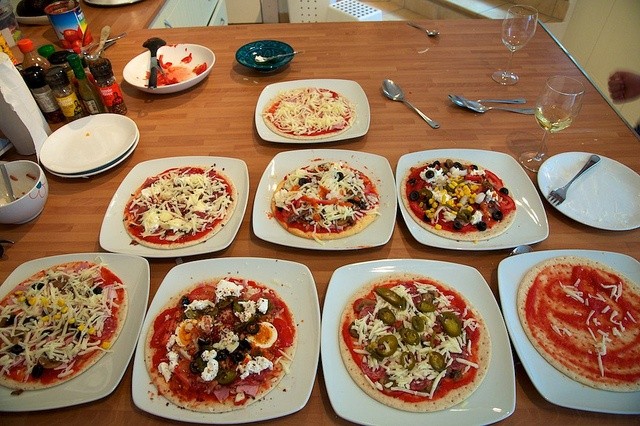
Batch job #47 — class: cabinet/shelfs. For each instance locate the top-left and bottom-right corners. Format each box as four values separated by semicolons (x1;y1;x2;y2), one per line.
1;0;226;28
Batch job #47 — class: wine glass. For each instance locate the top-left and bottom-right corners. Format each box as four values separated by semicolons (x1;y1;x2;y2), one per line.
492;5;539;86
520;75;586;173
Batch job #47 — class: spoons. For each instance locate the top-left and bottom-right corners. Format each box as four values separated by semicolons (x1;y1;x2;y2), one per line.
255;50;305;63
381;78;439;133
87;40;115;60
447;92;528;109
142;37;165;90
84;26;111;61
407;20;442;37
463;98;534;115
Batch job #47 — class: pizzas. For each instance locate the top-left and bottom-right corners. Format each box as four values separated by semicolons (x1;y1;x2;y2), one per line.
272;161;380;241
516;255;635;395
261;88;357;139
1;261;128;389
144;276;296;414
124;166;238;250
338;274;491;414
400;160;516;242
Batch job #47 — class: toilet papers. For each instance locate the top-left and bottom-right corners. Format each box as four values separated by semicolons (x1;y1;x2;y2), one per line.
0;54;49;155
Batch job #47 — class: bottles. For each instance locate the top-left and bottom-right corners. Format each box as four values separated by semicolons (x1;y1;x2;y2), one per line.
37;44;56;60
68;52;104;113
50;50;73;81
22;65;64;124
45;66;86;123
0;32;23;73
89;58;128;116
17;38;50;73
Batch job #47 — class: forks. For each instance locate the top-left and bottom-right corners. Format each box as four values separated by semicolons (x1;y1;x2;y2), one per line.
547;154;599;206
66;34;126;53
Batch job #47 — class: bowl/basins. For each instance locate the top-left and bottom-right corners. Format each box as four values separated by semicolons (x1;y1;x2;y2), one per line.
0;160;49;225
236;40;296;72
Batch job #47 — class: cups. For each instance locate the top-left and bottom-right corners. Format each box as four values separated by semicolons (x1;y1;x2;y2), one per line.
44;1;91;50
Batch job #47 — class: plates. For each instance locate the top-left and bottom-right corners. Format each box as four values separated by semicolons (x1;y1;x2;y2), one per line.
39;112;140;180
322;258;515;426
252;148;397;255
123;44;215;95
254;78;371;145
396;149;548;252
133;257;322;423
537;149;640;233
98;154;250;258
0;253;149;412
497;250;640;413
